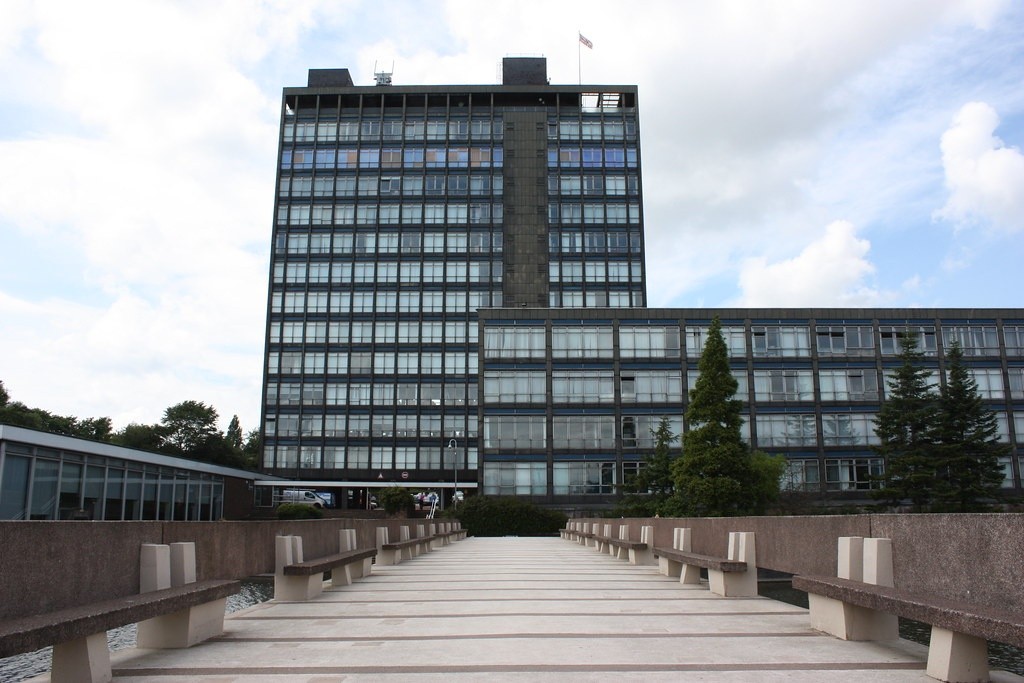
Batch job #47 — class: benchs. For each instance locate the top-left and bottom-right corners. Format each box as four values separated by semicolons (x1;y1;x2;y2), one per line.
376;524;437;565
429;522;469;547
592;524;655;566
274;529;378;599
652;527;758;597
0;543;241;683
792;537;1024;683
559;522;598;547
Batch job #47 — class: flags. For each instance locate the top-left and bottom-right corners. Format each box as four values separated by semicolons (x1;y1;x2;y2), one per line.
581;34;592;48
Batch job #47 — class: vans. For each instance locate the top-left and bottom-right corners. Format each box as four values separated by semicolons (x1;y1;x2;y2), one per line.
282;490;328;510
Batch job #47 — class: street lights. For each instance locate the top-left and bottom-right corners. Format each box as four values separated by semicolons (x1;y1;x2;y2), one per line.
448;438;460;513
